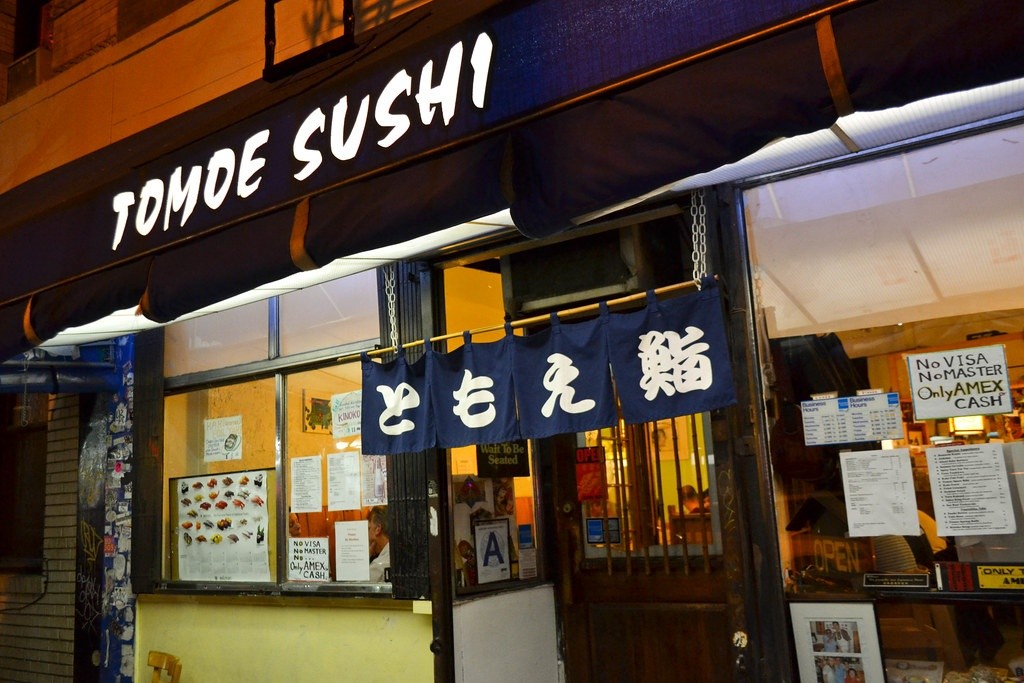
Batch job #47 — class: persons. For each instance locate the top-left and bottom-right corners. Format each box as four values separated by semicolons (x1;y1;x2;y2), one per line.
681;484;711;514
769;410;1006;667
366;505;389;582
458;541;477;586
288;506;301;538
495;478;513;515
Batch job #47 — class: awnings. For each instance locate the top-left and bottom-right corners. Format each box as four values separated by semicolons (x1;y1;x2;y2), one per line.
0;0;1024;363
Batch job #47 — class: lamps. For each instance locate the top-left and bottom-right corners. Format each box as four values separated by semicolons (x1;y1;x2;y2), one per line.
948;415;983;435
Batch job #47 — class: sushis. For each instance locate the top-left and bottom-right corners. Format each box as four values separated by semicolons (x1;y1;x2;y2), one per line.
181;475;264;545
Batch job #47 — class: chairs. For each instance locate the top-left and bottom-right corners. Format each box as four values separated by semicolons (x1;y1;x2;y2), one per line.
147;650;182;683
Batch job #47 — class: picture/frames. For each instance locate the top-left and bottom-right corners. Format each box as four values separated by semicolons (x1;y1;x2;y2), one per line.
302;388;336;435
788;599;888;683
